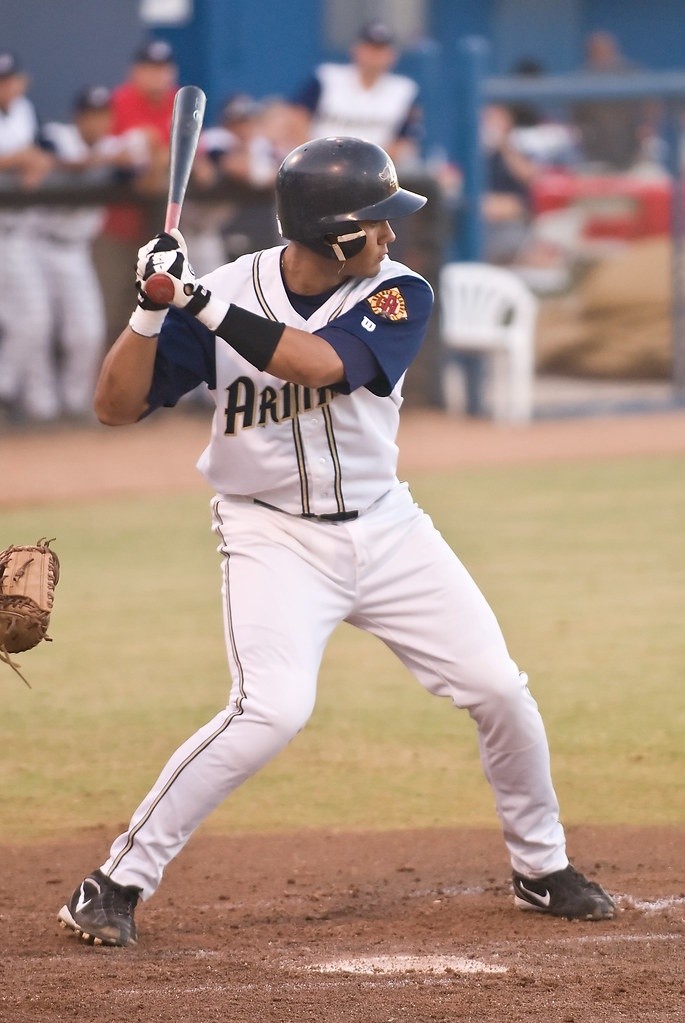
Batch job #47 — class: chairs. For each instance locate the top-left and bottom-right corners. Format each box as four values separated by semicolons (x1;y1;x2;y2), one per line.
440;260;537;423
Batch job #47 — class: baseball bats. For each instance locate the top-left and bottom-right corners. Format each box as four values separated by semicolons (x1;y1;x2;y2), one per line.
144;83;206;304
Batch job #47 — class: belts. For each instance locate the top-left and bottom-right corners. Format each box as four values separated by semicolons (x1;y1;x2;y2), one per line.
252;497;358;522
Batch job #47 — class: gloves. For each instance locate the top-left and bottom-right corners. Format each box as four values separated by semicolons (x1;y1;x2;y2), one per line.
128;227;285;373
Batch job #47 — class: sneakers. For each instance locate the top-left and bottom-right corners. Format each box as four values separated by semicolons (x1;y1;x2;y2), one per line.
511;864;616;921
56;869;144;949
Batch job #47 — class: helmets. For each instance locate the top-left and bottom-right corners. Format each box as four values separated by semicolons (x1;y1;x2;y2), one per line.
272;135;428;262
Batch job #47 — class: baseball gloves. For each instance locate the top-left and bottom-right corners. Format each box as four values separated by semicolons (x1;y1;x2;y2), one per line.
0;537;61;692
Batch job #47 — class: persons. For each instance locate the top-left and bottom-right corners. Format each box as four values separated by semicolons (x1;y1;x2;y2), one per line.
56;136;617;948
1;21;671;417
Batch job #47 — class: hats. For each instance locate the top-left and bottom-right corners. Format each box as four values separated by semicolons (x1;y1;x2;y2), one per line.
73;82;113;113
134;40;175;66
356;19;397;47
0;52;21;79
